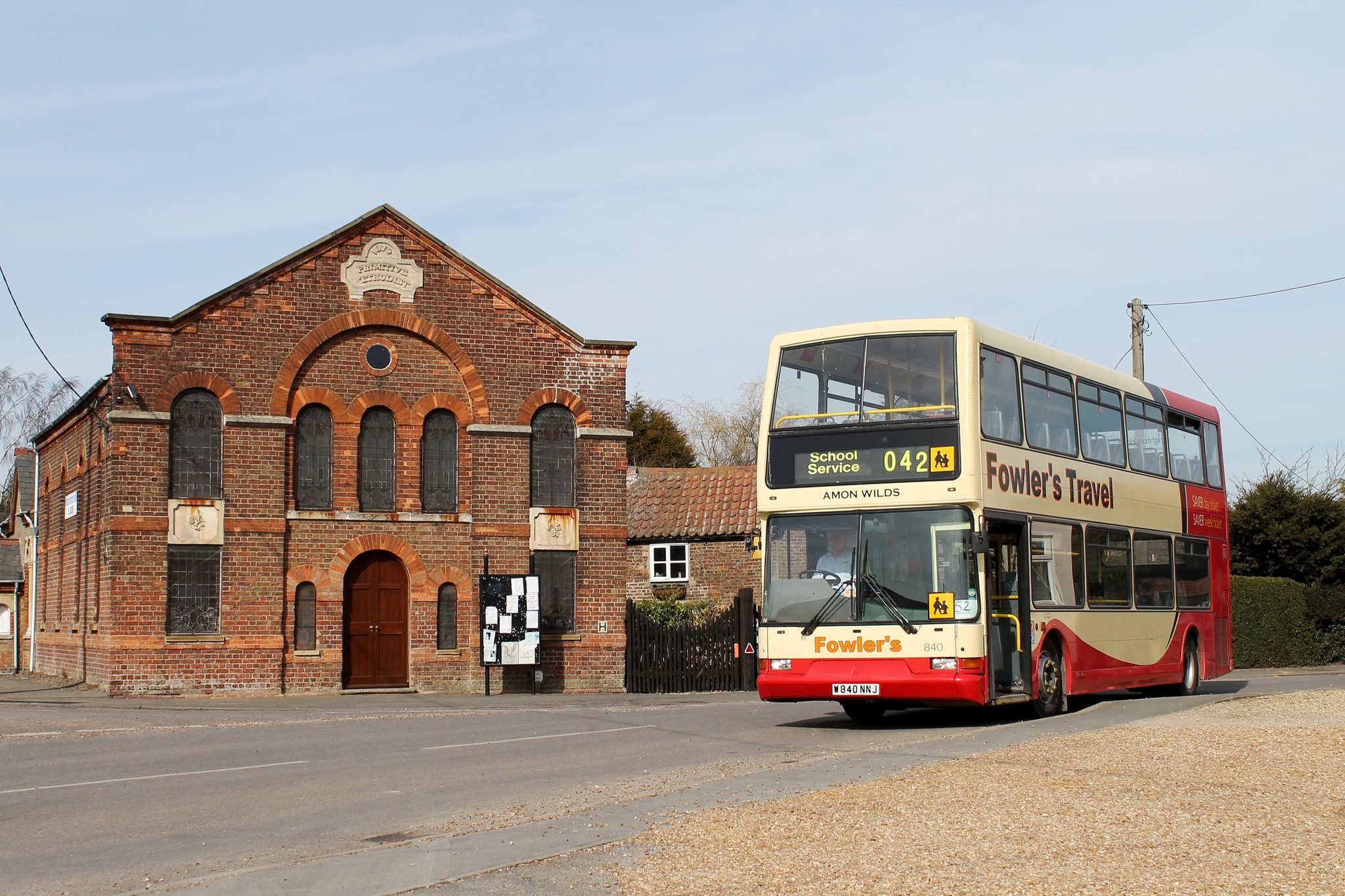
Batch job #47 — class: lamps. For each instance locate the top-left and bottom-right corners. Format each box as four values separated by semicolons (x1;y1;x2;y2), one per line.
116;383;137;403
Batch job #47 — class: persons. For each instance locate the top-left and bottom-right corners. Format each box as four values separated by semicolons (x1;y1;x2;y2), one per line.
810;526;858;598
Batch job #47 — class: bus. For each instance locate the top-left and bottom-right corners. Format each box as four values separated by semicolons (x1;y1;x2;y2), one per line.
756;316;1233;717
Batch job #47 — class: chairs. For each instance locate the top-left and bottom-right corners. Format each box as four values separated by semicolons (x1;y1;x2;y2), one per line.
1090;435;1110;462
809;405;958;428
1071;433;1091;457
1049;429;1071;452
1159;452;1177;476
1001;411;1021;441
1128;443;1145;470
1143;447;1162;474
1026;423;1049;447
981;404;1004;437
1171;454;1191;480
1188;459;1202;482
1108;439;1124;465
1207;466;1220;486
